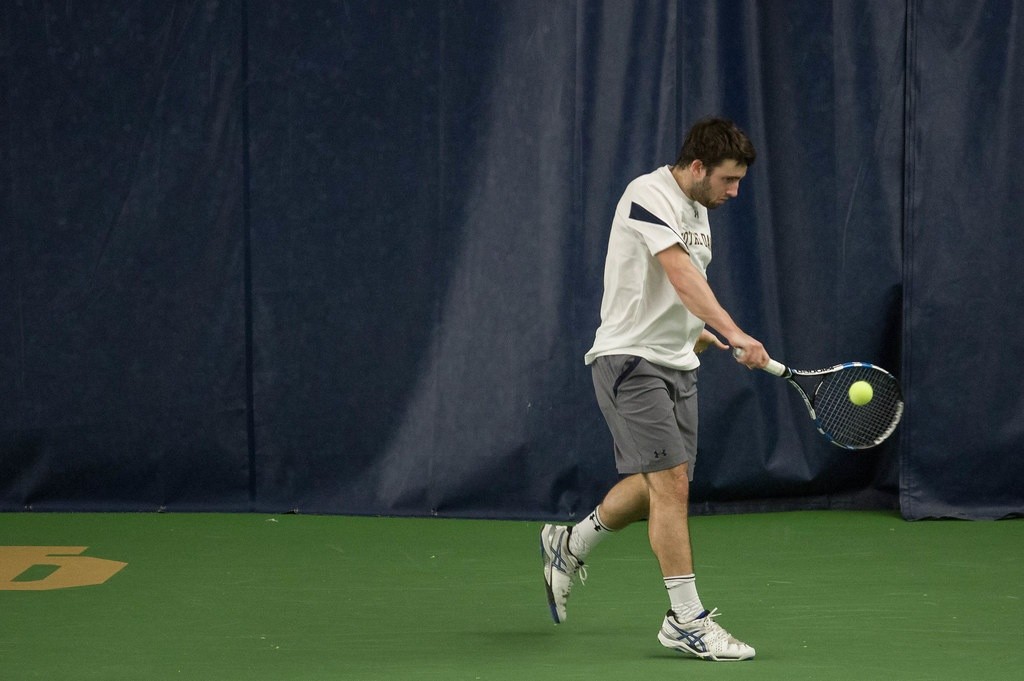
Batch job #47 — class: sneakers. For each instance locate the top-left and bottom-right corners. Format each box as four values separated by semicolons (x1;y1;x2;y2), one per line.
540;522;591;624
658;609;757;661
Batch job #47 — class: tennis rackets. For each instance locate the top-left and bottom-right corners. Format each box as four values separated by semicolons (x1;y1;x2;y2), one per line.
734;348;905;452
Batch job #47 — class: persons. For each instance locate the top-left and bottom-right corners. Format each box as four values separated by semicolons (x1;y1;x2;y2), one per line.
539;116;771;663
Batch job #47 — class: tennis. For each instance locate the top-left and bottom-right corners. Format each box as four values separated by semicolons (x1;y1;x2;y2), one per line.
849;381;873;405
